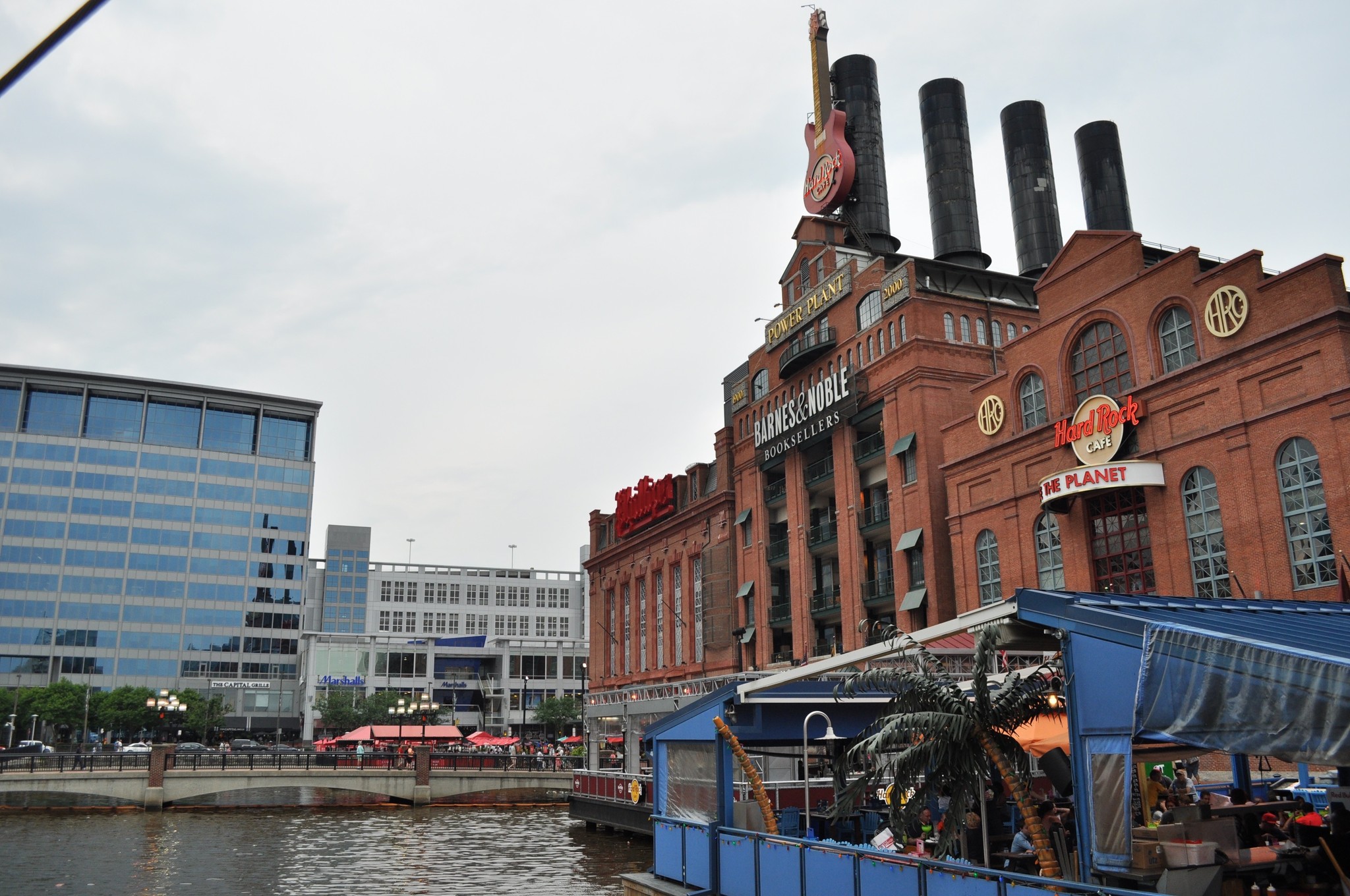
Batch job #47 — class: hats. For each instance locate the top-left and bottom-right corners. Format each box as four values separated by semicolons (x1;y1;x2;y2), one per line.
358;740;362;745
1153;765;1162;771
1176;769;1185;774
1160;810;1173;823
1261;813;1280;826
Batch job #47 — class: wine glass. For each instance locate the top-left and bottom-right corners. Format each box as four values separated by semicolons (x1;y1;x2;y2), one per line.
922;826;932;843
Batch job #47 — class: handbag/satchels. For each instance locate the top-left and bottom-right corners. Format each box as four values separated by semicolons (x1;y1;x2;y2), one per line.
870;827;904;851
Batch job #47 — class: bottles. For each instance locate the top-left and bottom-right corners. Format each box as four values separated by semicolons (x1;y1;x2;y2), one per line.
1251;882;1260;896
1267;883;1276;896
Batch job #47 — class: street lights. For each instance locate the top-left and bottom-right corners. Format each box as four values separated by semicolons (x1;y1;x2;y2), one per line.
409;693;440;745
620;720;628;774
9;674;22;748
275;670;284;751
203;679;211;745
146;688;179;744
451;672;456;727
801;710;848;837
30;714;38;745
82;666;94;752
159;695;186;744
388;699;415;746
8;714;17;748
586;723;591;770
522;675;529;736
428;681;433;702
578;663;587;740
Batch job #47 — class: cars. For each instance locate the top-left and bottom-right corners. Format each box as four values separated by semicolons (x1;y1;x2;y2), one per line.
445;744;462;753
269;744;301;752
471;743;498;754
176;742;216;752
117;743;152;754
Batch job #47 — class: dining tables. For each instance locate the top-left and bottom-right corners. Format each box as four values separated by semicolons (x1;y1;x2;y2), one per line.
1091;783;1333;896
774;797;1076;877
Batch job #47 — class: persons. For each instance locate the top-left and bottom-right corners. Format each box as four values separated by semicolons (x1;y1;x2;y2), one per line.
72;744;84;771
92;738;122;752
1004;789;1076;874
609;743;622;768
1252;795;1350;896
219;736;235;752
904;772;999;865
140;737;152;743
356;739;364;770
1147;755;1256;848
395;744;414;770
162;736;177;743
506;741;577;772
433;745;503;754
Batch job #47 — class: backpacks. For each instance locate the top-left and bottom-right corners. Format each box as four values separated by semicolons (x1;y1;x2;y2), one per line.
404;748;408;756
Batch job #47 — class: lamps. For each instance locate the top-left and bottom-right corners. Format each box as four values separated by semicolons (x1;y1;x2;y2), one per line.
721;381;740;385
753;385;763;390
724;703;737;724
1042;676;1064;709
803;712;847;836
754;265;897;321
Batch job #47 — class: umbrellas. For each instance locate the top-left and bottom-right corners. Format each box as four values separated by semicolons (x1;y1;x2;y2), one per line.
911;712;1212;764
313;730;519;748
556;735;643;742
255;731;285;741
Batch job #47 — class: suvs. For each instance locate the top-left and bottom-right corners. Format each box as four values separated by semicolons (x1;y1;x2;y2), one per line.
232;740;268;752
18;740;56;754
